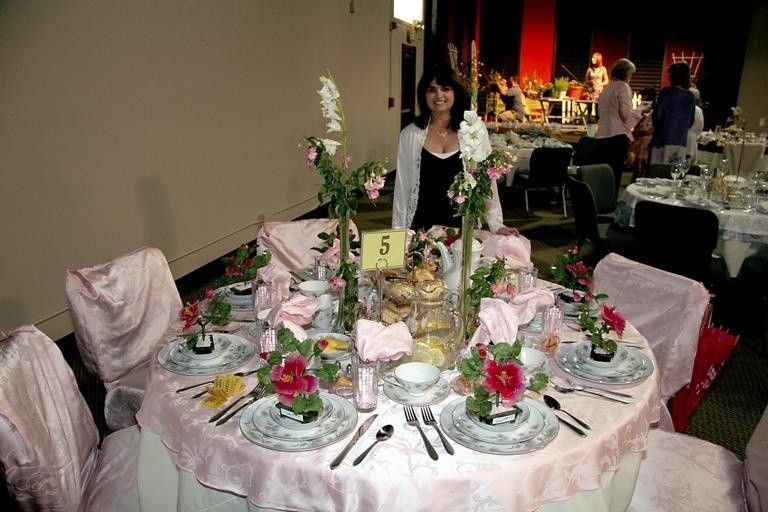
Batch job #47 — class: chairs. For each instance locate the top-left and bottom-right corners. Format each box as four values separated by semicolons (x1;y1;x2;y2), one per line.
428;226;531;271
3;324;142;511
258;217;360;279
592;253;711;436
65;245;186;431
484;122;766;319
625;406;767;510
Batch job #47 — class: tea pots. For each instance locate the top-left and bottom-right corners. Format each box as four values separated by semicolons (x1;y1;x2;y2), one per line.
432;229;483;289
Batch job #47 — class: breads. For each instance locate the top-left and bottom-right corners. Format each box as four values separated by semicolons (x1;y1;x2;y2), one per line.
381;260;448;332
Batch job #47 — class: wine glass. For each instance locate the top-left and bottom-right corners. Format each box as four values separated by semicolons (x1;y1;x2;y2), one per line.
636;156;767;214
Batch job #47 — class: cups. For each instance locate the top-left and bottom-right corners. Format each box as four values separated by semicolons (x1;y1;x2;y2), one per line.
251;277;272;315
315;258;328;281
381;362;441;398
255;318;278;362
517;266;538;293
543;303;565;340
350;363;379;412
328;367;355;399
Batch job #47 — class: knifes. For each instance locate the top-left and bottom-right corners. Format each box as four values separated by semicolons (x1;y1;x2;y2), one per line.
328;412;379;470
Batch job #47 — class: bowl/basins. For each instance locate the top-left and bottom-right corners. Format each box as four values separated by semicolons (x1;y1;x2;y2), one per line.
298;280;329;296
465;396;532;432
518;346;546;371
271;399;333;430
177;336;232;359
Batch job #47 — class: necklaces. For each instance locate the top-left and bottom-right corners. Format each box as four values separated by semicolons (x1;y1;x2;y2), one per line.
430;124;453;138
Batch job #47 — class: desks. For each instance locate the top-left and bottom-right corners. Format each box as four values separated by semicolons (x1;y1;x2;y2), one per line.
575;100;600;124
540;98;571;125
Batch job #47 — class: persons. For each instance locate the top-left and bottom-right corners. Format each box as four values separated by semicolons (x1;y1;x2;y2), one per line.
391;65;522;237
583;51;704;209
496;75;526;123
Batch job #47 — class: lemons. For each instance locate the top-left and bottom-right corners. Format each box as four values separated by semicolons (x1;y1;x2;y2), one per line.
407;317;452;367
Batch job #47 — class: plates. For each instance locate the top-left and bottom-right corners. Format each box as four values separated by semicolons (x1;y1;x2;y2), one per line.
552;288;601;320
215;281;254;307
441;390;560;458
380;376;450;405
238;391;358;454
254;397;345;440
452;400;546;446
553;341;655;387
169;335;246;369
157;332;255;377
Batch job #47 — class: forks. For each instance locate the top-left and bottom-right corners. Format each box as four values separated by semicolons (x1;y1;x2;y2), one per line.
547;378;633;409
207;381;269;429
401;403;438;462
419;403;453;457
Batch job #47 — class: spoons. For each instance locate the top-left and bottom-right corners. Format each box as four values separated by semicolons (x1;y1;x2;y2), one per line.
352;423;394;466
541;394;593;432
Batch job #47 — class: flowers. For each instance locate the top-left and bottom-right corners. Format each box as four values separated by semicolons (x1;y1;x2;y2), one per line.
224;241;273;281
258;323;343;422
538;77;593;100
464;233;533;310
446;109;519;219
456;337;549;419
574;298;628;357
297;61;389;214
176;288;232;350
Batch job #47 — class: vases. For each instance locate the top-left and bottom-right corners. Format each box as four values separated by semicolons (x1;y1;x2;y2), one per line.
338;212;350;263
457;216;476;332
